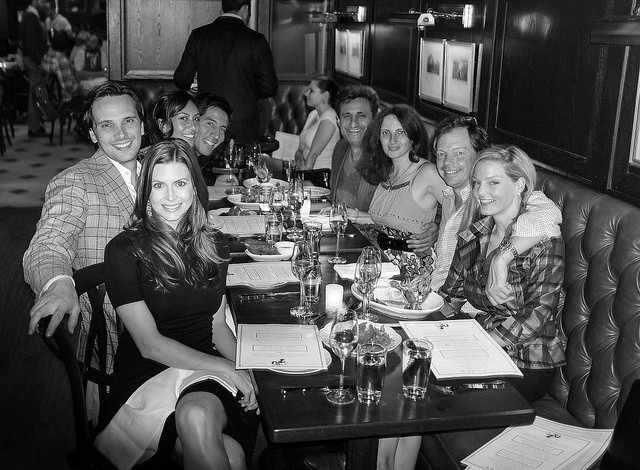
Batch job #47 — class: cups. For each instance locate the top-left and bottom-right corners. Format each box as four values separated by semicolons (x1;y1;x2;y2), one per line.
264;211;284;245
302;220;322;259
256;185;272;204
282;159;297;182
271;186;285;203
240;188;258;203
300;260;322;303
401;338;434;402
356;342;388;406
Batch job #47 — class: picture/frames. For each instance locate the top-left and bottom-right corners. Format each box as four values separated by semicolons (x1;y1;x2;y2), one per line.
418;36;445;104
347;30;364;79
334;28;348;74
442;40;476;116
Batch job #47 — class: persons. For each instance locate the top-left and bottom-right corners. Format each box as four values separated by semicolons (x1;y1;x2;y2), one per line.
429;115;564;316
192;93;232;160
173;0;278;140
22;81;145;393
377;145;569;470
1;0;104;138
93;138;261;470
355;104;449;282
330;85;386;214
138;91;200;165
294;75;342;171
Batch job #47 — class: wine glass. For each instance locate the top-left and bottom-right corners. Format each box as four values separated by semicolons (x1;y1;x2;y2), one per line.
325;308;360;405
286;183;305;240
327;201;348;264
290;240;316;319
245;141;262;167
358;245;381;321
354;255;379;320
224;144;238;182
286;178;304;233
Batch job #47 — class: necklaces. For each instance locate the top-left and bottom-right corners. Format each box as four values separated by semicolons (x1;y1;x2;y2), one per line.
387;156;419;192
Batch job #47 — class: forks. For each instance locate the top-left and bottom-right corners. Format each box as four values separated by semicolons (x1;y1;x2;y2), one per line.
427;382;507;396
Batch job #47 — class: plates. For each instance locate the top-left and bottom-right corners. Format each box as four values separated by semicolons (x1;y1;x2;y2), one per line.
301;215;333;232
350;279;445;319
245;248;290;261
226;193;260;210
268;348;332;375
303;186;331;199
207;207;257;216
319;319;403;358
242;178;290;192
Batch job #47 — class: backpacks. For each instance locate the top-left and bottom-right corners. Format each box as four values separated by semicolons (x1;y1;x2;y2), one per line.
31;70;63;122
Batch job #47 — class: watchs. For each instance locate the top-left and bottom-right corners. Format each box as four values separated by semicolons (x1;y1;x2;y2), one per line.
500;238;519;258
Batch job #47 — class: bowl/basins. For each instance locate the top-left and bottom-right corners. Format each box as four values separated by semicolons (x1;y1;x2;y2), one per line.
274;241;295;260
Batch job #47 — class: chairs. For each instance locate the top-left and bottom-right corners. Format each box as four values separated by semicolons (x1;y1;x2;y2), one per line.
35;263;118;470
0;65;104;155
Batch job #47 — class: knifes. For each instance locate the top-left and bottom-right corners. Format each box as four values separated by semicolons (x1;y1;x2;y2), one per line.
281;384;355;395
238;292;300;302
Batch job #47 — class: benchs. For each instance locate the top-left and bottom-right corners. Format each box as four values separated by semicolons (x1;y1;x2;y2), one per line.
436;168;638;465
124;78;313;140
